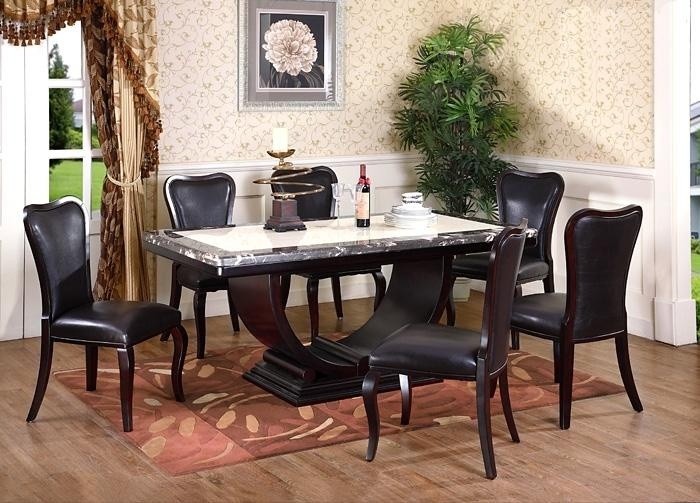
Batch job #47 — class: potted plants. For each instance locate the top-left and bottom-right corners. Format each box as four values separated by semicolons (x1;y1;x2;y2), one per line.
385;15;524;304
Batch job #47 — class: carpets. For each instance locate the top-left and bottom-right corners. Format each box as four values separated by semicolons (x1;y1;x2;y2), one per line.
53;333;626;477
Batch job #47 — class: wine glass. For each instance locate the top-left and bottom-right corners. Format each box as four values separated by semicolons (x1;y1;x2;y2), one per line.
332;184;355;227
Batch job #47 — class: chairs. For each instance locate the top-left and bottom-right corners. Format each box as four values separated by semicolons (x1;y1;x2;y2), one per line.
270;166;386;341
509;205;644;429
360;216;529;479
160;174;239;359
442;168;564;350
23;196;187;431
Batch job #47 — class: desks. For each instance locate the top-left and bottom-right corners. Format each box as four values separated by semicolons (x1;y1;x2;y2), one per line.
144;212;537;406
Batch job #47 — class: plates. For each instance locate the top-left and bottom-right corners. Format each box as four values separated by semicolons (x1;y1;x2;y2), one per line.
383;206;438;228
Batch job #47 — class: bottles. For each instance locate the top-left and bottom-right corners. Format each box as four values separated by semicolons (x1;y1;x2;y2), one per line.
355;163;370;227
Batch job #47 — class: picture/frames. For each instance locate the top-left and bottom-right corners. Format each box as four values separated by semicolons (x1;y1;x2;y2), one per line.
237;1;346;111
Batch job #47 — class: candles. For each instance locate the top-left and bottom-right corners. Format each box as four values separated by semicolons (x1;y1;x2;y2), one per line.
272;127;289;152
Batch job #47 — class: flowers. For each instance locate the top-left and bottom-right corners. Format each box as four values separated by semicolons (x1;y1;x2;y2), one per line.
259;19;324;88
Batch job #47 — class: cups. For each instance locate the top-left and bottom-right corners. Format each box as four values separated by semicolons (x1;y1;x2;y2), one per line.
401;192;423;207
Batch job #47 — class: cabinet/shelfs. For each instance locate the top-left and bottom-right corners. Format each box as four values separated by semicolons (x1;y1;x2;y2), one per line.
690;101;700;345
